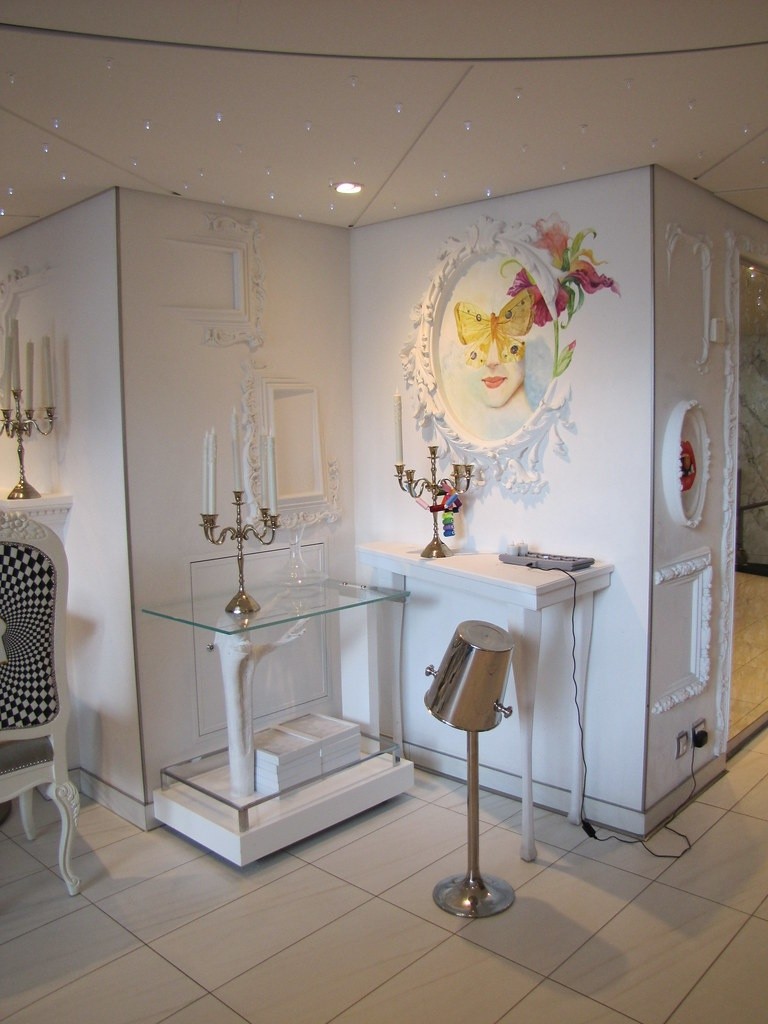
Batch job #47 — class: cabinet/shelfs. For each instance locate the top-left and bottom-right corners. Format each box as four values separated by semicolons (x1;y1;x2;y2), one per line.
140;576;419;866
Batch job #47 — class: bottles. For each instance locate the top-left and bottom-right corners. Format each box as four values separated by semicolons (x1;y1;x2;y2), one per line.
272;522;330;590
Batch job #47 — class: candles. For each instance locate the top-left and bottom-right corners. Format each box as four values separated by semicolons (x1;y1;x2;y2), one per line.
26;342;33;412
232;413;241;491
208;433;217;513
10;318;21;392
392;393;405;465
43;336;54;407
259;435;268;508
202;436;210;514
1;337;13;412
267;436;278;517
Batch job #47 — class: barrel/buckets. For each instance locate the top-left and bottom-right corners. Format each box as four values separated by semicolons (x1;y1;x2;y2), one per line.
423;620;515;732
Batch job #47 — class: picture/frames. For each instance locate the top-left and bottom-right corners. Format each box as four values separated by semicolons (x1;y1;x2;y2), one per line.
243;365;342;530
163;214;265;354
397;213;578;491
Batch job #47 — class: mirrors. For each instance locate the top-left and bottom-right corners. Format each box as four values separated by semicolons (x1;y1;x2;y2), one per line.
720;254;766;757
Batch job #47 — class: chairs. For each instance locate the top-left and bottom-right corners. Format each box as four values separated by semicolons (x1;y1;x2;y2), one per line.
0;509;79;896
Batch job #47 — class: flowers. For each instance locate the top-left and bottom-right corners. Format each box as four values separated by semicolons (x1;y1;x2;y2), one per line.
500;210;623;382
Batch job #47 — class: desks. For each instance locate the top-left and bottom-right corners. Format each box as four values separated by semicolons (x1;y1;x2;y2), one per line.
2;488;73;542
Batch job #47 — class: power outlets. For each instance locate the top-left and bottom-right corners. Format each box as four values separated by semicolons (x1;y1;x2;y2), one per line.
692;716;707;736
